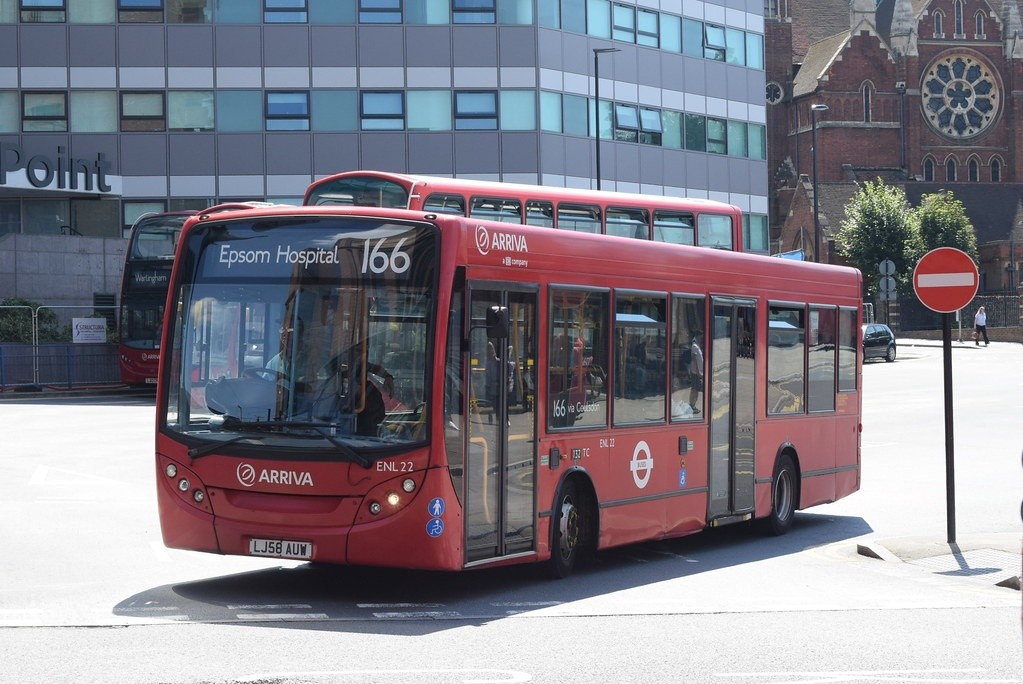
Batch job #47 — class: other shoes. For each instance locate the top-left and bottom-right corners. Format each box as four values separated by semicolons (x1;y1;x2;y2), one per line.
692;407;700;413
985;341;989;344
976;343;979;345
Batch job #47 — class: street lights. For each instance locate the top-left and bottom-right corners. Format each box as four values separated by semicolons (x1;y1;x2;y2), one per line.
811;104;829;264
594;48;623;190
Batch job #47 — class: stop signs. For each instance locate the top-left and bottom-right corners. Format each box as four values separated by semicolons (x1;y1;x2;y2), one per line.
912;247;979;313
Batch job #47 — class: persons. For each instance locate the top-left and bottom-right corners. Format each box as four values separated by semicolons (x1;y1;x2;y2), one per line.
689;330;704;413
446;328;691;451
483;342;514;427
973;306;990;346
263;314;318;400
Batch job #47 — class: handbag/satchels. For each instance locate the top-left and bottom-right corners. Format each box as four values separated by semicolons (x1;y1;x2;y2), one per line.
972;329;978;339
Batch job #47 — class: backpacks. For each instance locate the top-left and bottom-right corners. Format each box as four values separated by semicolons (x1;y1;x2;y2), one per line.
682;349;692;363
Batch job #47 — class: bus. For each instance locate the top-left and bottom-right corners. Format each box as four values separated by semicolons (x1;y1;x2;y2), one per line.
277;171;743;414
119;210;266;384
155;202;862;577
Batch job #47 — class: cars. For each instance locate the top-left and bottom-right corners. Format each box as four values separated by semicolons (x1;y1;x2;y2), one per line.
861;323;896;363
